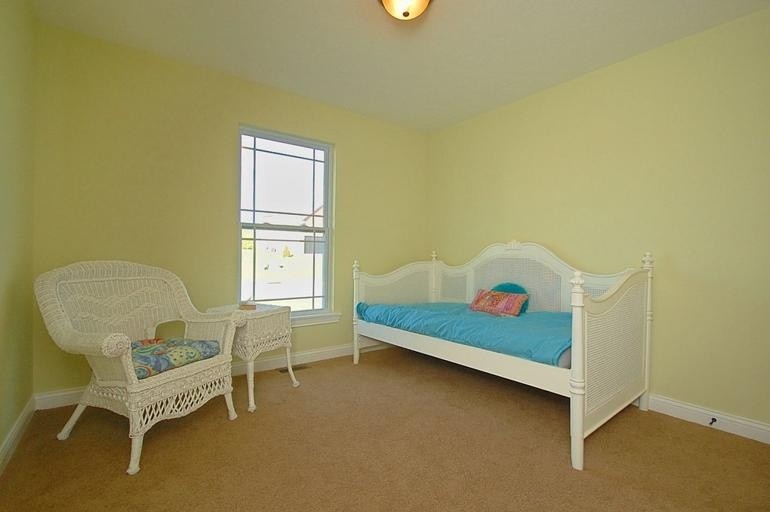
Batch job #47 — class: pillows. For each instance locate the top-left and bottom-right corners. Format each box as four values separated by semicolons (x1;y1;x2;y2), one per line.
469;288;529;318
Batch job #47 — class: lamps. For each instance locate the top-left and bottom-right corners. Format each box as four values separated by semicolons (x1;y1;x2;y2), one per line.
381;0;431;21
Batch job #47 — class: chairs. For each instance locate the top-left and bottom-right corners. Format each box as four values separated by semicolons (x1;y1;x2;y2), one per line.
33;260;248;476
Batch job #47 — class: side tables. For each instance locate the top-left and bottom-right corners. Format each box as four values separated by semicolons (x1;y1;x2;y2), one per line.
206;302;300;413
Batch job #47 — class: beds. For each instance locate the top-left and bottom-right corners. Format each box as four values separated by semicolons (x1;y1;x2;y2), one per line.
352;242;655;471
